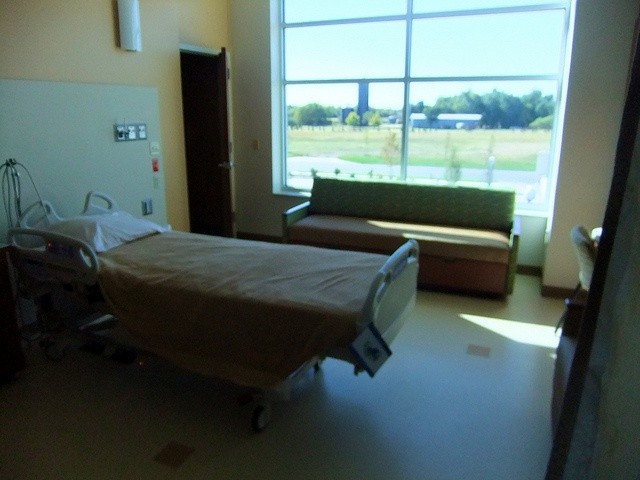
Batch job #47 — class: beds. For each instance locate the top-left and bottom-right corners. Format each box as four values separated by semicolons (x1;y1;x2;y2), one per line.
7;193;420;430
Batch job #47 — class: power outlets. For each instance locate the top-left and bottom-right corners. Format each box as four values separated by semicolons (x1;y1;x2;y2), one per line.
139;126;146;139
127;125;136;140
114;124;125;140
150;142;160;156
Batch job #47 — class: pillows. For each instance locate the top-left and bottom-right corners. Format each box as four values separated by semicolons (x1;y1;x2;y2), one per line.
50;209;172;252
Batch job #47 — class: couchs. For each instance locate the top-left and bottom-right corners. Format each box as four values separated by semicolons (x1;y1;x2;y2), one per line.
283;177;519;300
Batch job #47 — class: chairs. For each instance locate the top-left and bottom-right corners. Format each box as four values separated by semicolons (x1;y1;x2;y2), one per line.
555;224;597;334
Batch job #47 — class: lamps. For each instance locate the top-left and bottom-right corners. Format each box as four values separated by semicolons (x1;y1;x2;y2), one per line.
117;1;142;52
